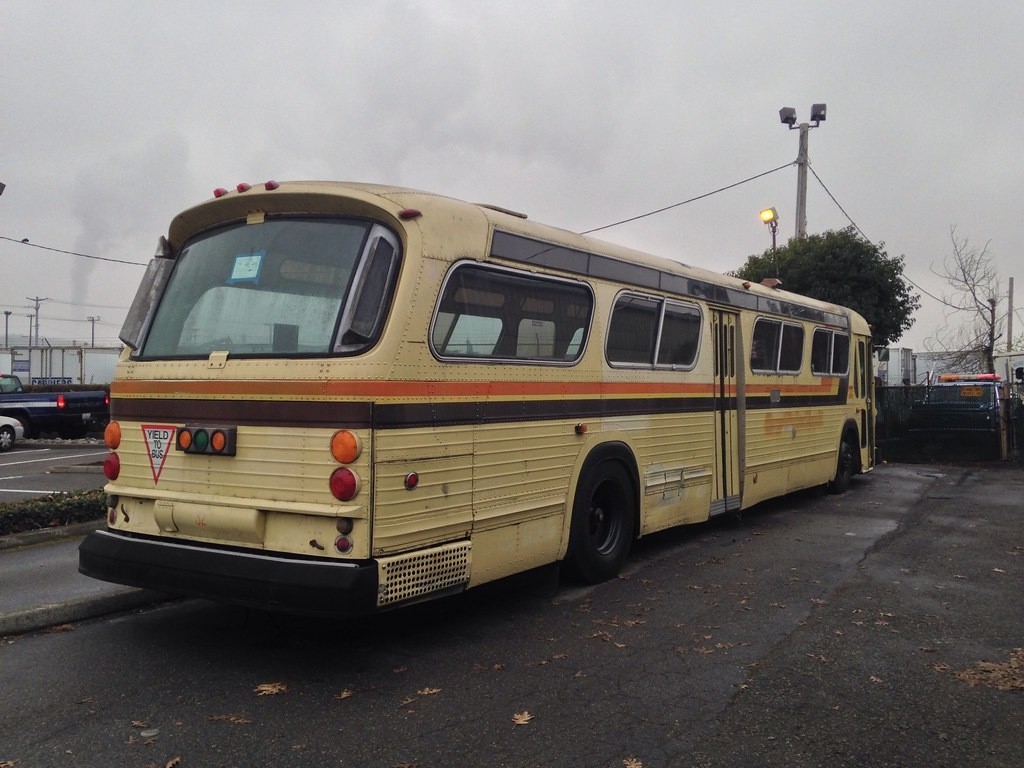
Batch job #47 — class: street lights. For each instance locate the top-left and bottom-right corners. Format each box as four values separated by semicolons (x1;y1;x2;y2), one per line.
777;102;827;242
760;205;781;292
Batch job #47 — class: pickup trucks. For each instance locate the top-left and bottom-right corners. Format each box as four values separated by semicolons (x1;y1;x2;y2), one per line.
0;373;109;442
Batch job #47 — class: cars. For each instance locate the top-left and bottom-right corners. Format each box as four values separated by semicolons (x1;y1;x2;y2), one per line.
909;373;1020;435
0;416;25;452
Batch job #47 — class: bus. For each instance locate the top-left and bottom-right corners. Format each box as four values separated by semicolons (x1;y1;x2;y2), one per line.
70;179;878;616
